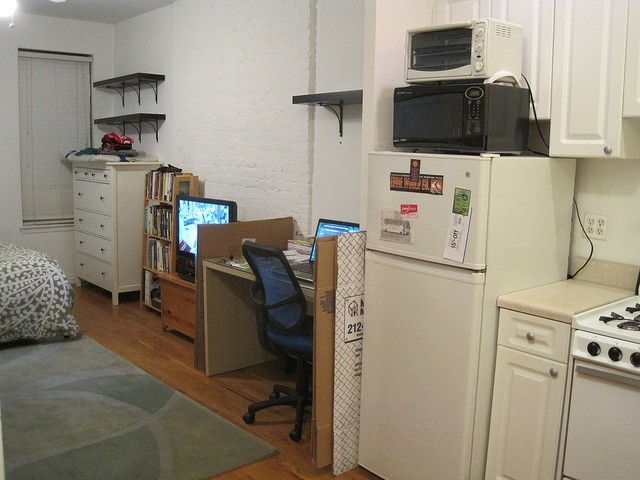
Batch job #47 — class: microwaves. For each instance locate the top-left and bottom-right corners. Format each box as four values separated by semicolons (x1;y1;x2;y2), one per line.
393;86;529;153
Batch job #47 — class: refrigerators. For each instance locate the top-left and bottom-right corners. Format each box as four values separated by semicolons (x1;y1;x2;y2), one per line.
358;151;576;480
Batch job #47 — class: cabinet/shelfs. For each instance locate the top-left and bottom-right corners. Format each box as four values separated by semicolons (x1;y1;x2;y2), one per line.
623;0;640;117
155;271;199;345
93;71;165;143
554;0;630;158
72;162;161;305
484;297;574;480
433;0;492;22
493;0;552;121
141;167;198;315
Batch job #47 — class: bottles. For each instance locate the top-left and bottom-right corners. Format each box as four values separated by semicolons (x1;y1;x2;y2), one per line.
242;237;255;268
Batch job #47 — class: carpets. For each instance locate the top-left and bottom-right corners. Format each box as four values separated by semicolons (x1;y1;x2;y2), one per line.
0;336;278;480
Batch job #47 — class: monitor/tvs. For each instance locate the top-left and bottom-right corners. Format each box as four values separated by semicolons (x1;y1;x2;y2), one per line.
175;194;238;284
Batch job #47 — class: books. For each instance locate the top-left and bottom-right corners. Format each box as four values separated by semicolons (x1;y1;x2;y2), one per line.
143;269;162;311
145;171;193;201
145;205;172;239
286;239;313;253
148;239;169;273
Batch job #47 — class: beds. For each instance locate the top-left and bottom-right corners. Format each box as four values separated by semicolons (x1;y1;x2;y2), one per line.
0;239;80;340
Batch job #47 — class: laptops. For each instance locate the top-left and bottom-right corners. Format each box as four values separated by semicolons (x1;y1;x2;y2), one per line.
285;219;362;284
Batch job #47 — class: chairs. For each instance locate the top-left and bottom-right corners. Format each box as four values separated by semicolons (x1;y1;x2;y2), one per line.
240;239;321;441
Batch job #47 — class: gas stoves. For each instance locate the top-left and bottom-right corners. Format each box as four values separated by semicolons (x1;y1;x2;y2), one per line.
570;294;640;375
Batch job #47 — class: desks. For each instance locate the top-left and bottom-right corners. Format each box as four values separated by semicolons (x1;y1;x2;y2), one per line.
202;237;333;398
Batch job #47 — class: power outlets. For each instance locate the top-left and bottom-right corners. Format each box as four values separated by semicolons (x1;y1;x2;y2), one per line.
584;214;607;241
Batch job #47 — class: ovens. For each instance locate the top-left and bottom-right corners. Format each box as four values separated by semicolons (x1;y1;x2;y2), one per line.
404;18;523;84
